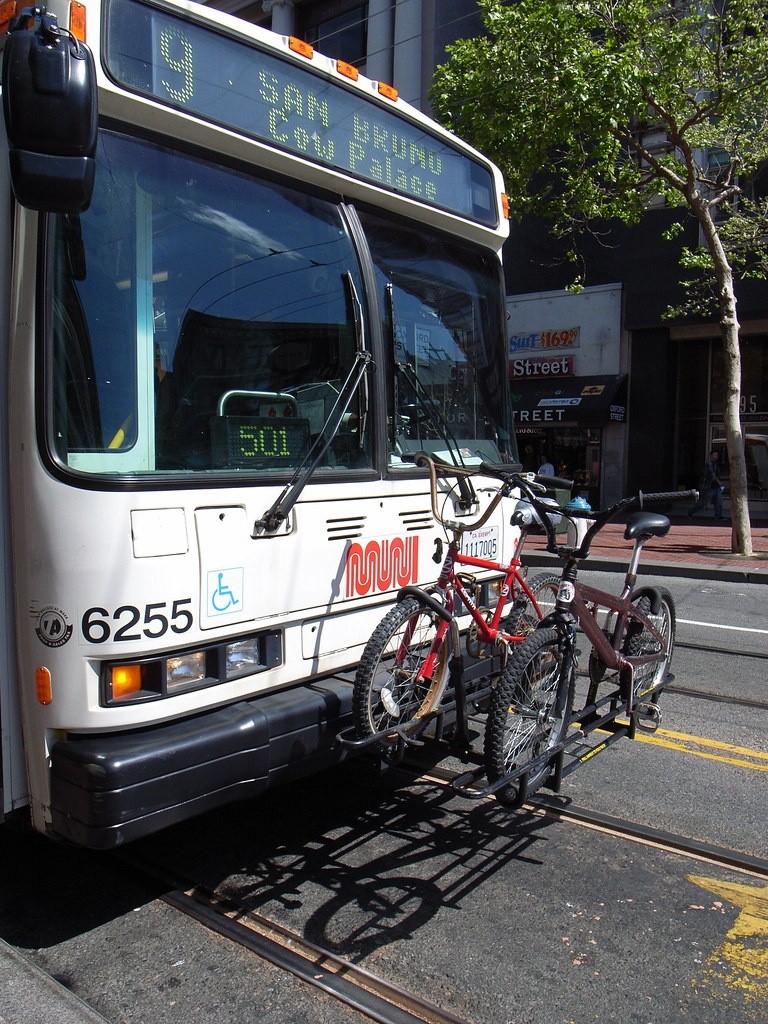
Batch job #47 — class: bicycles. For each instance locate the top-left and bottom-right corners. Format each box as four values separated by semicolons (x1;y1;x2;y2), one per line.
352;449;573;757
478;460;678;810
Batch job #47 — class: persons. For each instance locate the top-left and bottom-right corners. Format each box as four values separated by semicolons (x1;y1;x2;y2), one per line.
538;456;554;477
686;451;727;521
264;345;301;394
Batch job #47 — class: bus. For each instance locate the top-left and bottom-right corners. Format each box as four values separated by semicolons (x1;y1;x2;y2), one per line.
0;0;526;857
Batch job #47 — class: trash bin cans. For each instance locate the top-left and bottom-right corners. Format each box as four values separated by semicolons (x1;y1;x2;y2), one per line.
521;488;571;535
